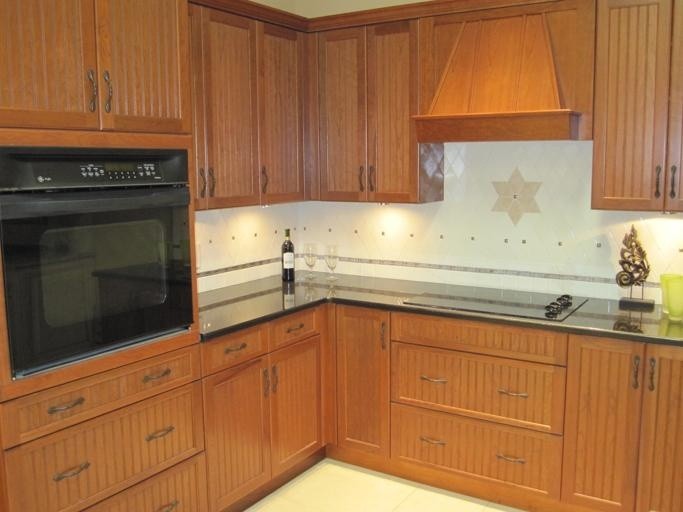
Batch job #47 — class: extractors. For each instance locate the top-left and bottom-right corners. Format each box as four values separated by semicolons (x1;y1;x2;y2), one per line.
411;110;581;142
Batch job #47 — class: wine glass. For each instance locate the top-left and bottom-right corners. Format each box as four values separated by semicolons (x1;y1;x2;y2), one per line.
305;279;318;302
304;244;317;279
326;281;338;298
325;246;339;281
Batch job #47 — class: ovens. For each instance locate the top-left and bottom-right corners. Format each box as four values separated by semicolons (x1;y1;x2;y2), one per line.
0;143;193;379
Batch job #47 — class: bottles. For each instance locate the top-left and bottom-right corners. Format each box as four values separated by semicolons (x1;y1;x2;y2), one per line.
282;282;295;311
281;229;295;282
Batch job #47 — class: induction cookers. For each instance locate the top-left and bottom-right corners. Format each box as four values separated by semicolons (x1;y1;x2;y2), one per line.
397;283;591;322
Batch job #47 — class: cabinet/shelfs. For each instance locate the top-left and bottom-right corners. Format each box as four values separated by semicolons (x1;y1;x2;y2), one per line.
328;297;390;475
558;328;683;512
0;334;209;512
201;298;328;512
0;0;191;136
261;4;311;207
311;0;443;205
192;0;260;211
390;305;568;512
591;0;683;212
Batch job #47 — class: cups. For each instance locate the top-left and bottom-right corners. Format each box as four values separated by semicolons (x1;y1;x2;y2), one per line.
667;322;681;339
660;274;674;313
667;276;683;321
657;312;667;337
